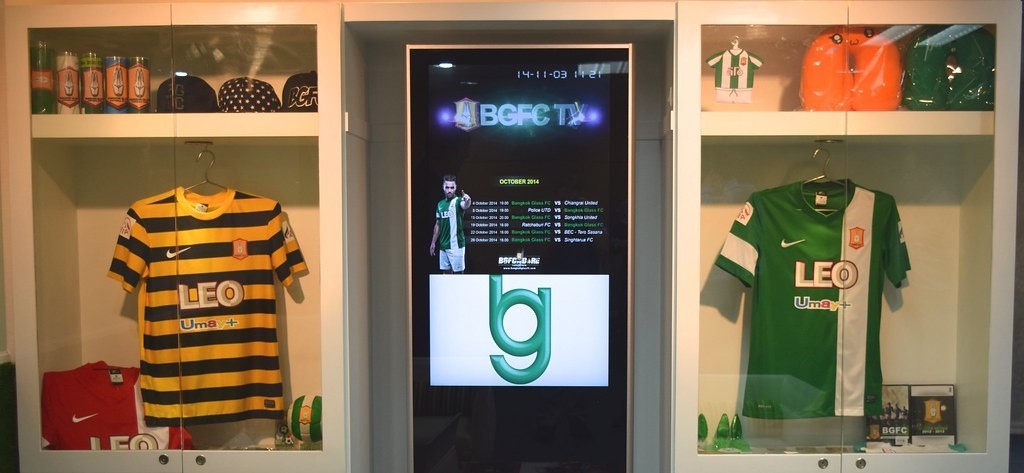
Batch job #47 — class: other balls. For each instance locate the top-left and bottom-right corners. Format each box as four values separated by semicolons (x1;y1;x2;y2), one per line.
288;394;323;442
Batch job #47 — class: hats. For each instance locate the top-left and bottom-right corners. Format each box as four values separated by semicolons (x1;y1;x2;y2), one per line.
278;70;317;113
218;76;281;112
158;75;218;112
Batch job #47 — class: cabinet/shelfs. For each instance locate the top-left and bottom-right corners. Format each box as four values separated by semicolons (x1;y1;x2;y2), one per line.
0;0;1014;472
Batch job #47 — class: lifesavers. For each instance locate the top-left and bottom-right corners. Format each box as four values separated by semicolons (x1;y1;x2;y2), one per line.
899;24;995;112
796;25;902;112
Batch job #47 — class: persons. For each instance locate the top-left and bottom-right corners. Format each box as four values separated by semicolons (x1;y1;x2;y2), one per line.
430;175;471;275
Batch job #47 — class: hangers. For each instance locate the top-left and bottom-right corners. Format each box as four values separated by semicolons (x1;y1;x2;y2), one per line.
802;147;848;213
184;150;230;209
